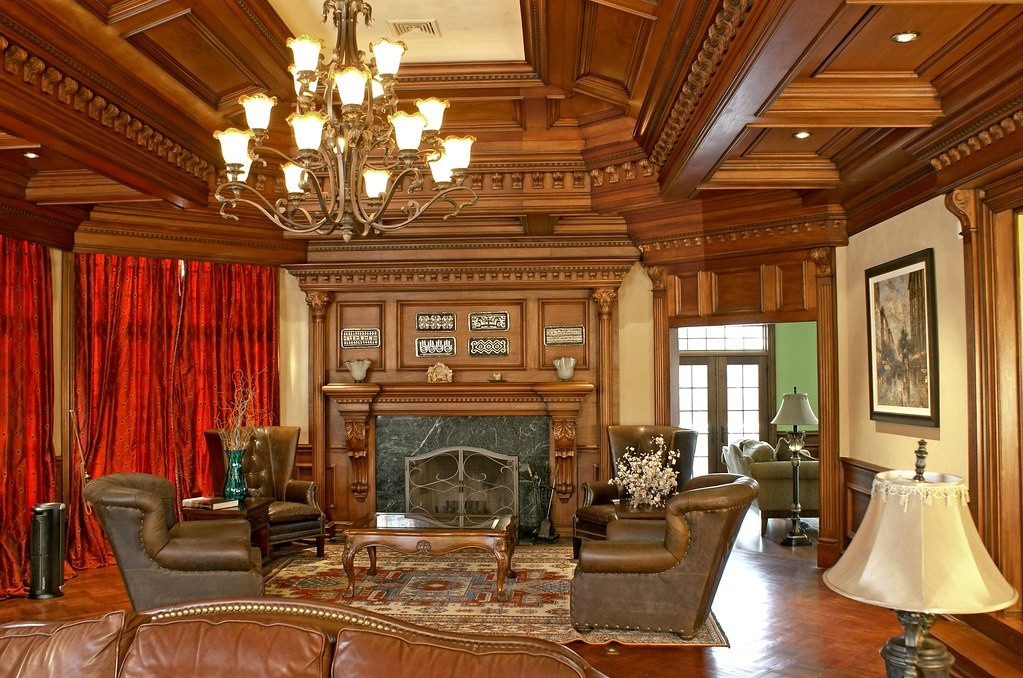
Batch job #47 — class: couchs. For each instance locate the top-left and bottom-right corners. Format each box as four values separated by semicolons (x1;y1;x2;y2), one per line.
570;473;761;641
83;472;264;613
573;425;698;558
204;425;327;557
0;597;609;678
722;438;815;537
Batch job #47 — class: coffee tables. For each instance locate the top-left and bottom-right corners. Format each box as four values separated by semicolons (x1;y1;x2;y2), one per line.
342;512;518;602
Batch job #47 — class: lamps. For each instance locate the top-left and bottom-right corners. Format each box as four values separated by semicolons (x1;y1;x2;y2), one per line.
769;386;819;547
823;440;1019;678
212;0;479;242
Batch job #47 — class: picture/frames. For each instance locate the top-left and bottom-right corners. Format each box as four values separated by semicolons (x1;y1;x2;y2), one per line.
864;248;940;428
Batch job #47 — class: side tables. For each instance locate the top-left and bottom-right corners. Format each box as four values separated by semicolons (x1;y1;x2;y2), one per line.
614;502;667;521
181;498;274;566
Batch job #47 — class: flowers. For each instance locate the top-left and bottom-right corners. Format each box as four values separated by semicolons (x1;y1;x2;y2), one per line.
607;433;681;511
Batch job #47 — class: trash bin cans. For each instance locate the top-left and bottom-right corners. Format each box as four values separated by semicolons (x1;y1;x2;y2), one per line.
29;502;66;599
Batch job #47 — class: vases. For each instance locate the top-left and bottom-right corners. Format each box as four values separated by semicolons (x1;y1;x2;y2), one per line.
223;449;247;501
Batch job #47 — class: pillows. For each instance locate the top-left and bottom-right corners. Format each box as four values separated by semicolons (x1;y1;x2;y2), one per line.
740;438;815;463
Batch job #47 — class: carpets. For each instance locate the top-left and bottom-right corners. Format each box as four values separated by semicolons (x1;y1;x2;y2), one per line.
264;543;732;648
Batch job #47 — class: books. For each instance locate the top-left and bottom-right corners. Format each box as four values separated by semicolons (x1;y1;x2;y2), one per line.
182;497;238;510
